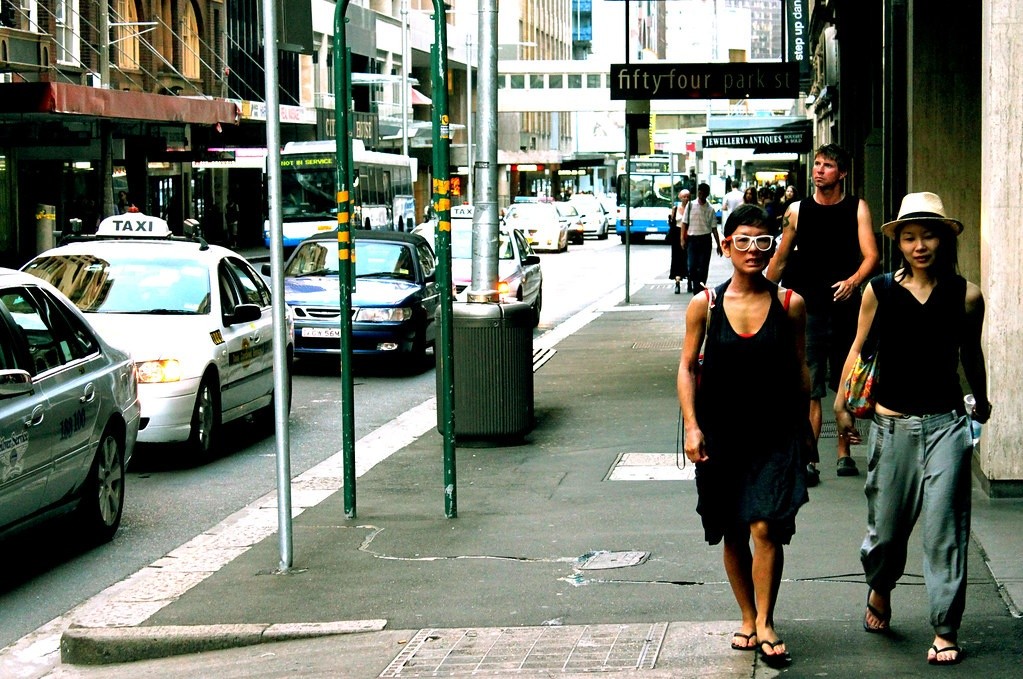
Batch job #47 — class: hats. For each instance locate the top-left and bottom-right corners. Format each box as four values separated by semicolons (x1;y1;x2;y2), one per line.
880;192;965;241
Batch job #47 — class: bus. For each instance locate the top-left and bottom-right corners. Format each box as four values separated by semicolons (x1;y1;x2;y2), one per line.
707;177;726;224
610;173;690;245
261;139;416;248
616;159;670;177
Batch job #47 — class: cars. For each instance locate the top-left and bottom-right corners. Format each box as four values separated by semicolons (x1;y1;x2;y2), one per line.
501;203;570;253
2;240;295;465
261;231;457;367
399;219;543;327
597;196;624;230
552;201;586;245
569;199;610;240
0;266;140;541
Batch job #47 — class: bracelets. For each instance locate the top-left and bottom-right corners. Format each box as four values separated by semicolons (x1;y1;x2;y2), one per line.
972;403;992;420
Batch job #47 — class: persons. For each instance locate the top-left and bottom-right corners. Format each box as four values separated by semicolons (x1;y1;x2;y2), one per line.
766;143;879;485
667;176;797;294
87;191;136;233
224;194;240;246
556;187;572;201
834;192;991;665
677;202;810;661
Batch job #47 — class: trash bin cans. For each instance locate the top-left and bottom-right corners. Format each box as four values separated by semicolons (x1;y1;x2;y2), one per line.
433;291;535;444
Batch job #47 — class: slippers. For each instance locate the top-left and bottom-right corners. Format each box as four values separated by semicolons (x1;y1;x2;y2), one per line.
731;625;758;651
929;636;962;665
862;586;892;633
756;633;792;668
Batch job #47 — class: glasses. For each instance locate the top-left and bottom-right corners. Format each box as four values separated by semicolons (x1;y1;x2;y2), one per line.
725;234;775;253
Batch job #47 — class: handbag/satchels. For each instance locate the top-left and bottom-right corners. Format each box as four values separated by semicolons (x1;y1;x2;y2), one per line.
665;206;677;245
683;201;692;250
844;271;894;418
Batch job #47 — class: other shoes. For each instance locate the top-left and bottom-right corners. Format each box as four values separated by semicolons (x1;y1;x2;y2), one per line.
687;281;692;292
806;464;819;489
675;282;681;295
837;455;856;475
